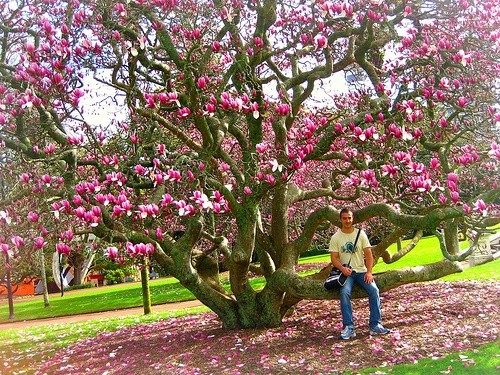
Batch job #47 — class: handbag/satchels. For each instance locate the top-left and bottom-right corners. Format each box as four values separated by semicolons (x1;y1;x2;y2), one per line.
324;264;351;290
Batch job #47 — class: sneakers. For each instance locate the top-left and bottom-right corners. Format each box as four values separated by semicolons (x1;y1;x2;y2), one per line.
342;326;356;339
370;325;390;335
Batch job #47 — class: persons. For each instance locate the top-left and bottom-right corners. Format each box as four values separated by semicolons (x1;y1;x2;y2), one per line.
329;208;391;340
